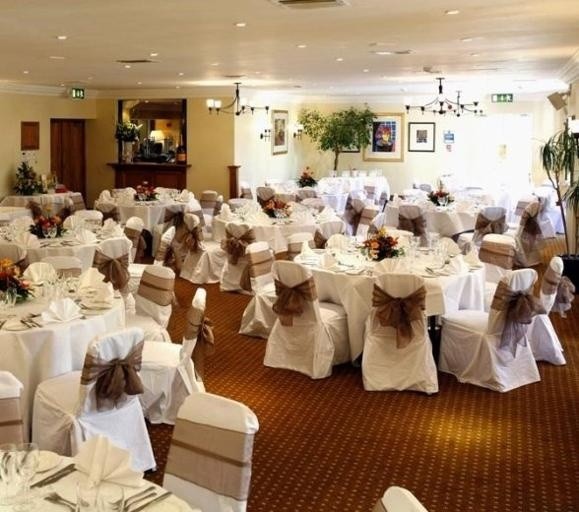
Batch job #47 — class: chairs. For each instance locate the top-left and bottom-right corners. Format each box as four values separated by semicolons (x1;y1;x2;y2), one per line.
29;327;157;480
237;242;278;339
0;371;26;448
1;169;578;293
360;273;439;395
162;390;260;511
135;287;215;426
124;264;176;342
528;257;568;366
261;259;352;379
436;267;541;394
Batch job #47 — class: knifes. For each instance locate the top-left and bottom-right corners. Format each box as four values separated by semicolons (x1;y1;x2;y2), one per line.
132;491;172;512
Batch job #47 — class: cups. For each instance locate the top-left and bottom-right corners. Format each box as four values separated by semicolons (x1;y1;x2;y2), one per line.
76;480;96;512
97;485;123;512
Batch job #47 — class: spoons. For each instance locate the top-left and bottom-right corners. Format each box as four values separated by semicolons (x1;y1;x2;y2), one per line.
112;486;156;510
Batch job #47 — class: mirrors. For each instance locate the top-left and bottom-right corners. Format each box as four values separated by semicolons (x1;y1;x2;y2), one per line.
118;98;187;165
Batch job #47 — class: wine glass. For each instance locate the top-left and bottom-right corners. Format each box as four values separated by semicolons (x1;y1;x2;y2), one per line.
13;443;40;512
41;225;57;242
7;287;17;318
403;232;449;268
438;196;449;209
43;271;73;301
0;444;16;506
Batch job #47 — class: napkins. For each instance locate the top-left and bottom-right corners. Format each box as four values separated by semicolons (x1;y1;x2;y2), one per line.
74;436;144;501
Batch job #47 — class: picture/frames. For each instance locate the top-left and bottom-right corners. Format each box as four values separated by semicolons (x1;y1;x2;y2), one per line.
335;124;361;153
362;113;405;162
408;122;436;153
271;110;289;155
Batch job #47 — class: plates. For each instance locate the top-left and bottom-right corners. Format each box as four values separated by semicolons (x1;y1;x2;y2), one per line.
35;450;61;473
3;321;32;331
345;269;366;275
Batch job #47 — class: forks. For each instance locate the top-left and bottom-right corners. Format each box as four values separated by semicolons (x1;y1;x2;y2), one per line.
49;492;76;507
123;493;156;512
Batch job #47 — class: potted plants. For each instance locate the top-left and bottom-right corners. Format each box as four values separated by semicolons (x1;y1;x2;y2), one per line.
115;120;144;164
540;118;579;296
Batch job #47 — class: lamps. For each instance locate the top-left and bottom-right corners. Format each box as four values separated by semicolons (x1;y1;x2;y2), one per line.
207;82;270;118
404;77;483;118
